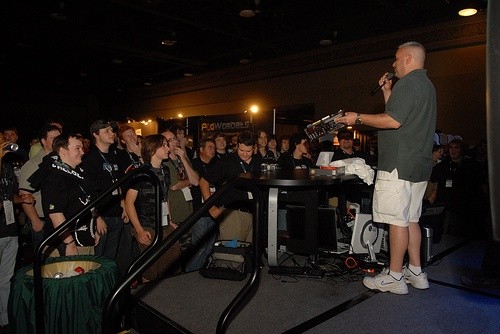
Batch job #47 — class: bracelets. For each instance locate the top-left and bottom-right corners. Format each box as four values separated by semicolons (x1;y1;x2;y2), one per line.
65;239;74;246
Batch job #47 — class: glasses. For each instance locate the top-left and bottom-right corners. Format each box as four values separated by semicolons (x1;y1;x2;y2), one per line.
166;136;177;142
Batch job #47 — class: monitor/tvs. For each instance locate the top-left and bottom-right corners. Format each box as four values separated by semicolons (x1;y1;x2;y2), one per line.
348;213;384;264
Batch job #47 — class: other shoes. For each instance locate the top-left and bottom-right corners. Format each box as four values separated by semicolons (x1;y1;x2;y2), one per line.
142;277;150;283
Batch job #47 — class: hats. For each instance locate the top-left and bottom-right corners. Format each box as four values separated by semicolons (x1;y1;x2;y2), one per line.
90;121;118;136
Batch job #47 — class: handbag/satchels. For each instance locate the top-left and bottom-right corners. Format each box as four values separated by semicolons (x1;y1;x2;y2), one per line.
198;240;253;281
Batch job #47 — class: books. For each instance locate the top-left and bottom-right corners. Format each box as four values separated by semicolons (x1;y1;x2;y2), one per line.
304;109;346;142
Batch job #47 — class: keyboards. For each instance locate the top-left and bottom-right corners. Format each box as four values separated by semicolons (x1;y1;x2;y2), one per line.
269;265;326;279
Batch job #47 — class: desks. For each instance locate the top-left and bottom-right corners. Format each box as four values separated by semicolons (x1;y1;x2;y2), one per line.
240;169;342;269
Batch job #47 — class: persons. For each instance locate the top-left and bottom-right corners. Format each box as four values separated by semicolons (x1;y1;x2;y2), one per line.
200;130;262;247
0;133;20;334
119;126;144;164
42;130;100;256
3;129;27;169
75;120;129;284
161;131;199;253
276;134;315;180
426;130;487;287
19;125;61;249
125;135;178;283
82;139;90;152
334;41;437;295
29;122;63;160
255;131;277;176
177;127;378;272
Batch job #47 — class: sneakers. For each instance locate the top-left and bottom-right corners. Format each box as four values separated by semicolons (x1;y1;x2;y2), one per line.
363;268;409;295
401;263;429;289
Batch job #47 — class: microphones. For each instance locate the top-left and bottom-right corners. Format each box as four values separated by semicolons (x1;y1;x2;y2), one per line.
369;73;394;97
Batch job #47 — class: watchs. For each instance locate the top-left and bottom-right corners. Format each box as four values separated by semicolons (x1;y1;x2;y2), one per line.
355;113;362;126
